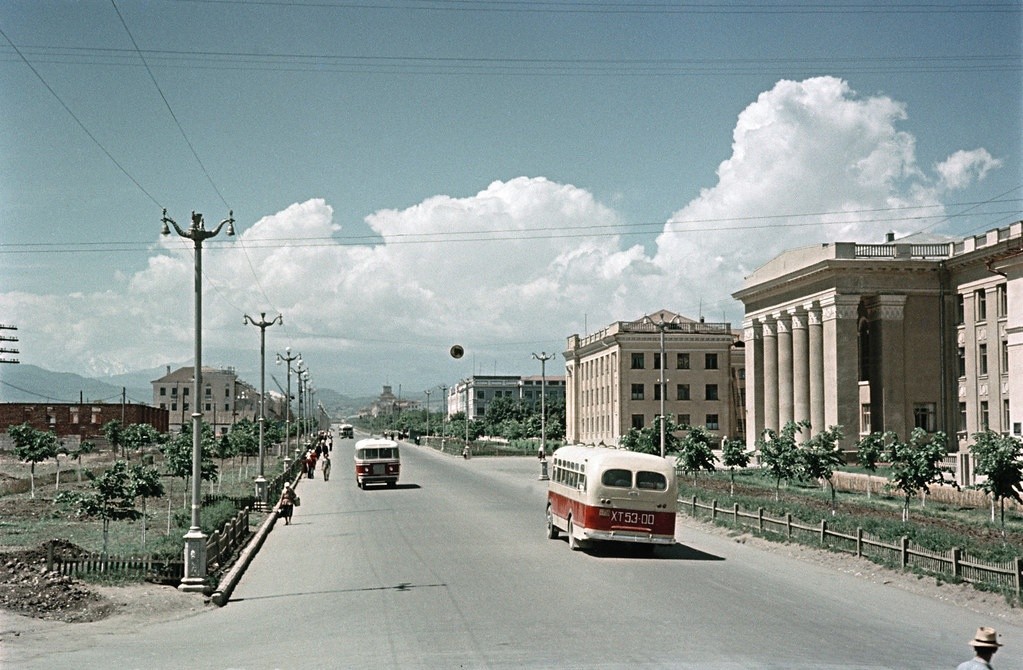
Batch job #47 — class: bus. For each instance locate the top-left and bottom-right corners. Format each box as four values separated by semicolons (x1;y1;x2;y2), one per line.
339;424;353;439
354;437;400;489
545;444;677;553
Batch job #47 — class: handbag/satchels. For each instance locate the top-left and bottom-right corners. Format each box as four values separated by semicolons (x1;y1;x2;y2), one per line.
294;496;301;506
276;507;284;518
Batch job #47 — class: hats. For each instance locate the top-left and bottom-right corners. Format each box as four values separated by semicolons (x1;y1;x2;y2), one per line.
968;626;1003;648
308;449;311;452
284;481;290;486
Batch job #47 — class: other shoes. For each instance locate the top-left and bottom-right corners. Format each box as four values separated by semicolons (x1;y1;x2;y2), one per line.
289;522;291;525
286;522;288;525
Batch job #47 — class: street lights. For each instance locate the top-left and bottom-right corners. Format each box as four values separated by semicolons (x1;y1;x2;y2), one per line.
242;312;283;509
641;311;682;458
530;350;556;454
290;362;331;458
438;383;451;451
275;345;303;474
424;389;435;445
461;376;475;446
159;207;236;595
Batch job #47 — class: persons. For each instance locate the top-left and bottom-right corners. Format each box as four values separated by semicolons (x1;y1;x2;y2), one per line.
955;626;1004;670
280;482;296;525
384;432;394;440
306;431;333;481
